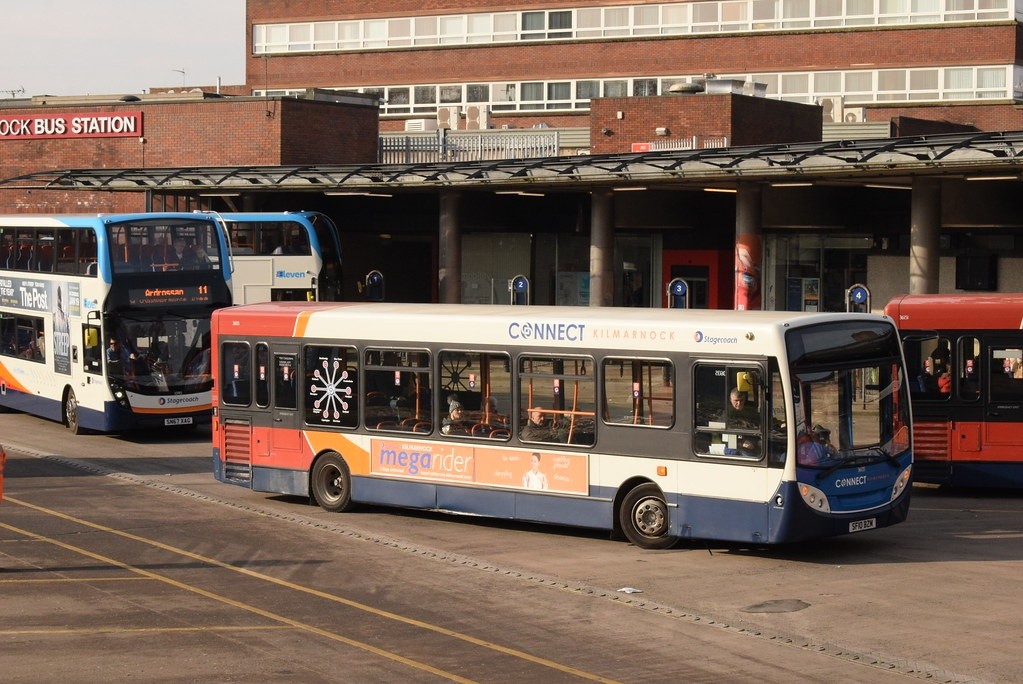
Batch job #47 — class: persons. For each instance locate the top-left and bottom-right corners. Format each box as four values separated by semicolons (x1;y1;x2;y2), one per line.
443;394;473;436
927;336;952;395
165;238;195;270
192;245;212;269
106;335;132;373
718;387;786;455
482;395;501;427
523;453;548;491
520;406;555;442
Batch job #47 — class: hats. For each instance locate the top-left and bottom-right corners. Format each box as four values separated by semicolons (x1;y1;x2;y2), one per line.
447;394;464;413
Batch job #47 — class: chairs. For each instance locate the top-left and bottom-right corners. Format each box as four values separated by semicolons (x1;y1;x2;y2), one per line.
342;392;511;439
0;242;186;277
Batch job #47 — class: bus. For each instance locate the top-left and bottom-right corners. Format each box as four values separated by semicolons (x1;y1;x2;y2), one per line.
885;293;1023;497
0;210;343;435
211;302;915;549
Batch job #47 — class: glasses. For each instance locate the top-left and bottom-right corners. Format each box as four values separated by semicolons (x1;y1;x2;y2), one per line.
110;343;118;345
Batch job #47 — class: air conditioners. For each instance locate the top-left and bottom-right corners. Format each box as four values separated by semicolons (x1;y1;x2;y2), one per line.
819;96;844;123
438;107;461;129
844;107;866;123
466;105;490;129
